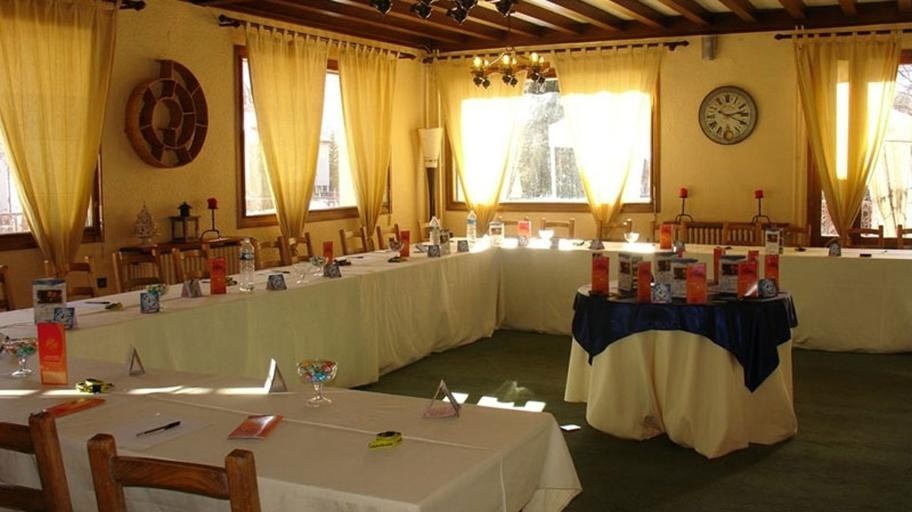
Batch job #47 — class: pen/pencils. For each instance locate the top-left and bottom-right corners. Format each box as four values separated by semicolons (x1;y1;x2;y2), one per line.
352;257;363;259
135;420;182;437
84;301;110;304
271;270;290;273
201;281;211;283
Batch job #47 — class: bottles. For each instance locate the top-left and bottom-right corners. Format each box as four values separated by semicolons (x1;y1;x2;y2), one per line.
428;213;441;246
465;209;477;245
237;237;255;291
439;228;450;256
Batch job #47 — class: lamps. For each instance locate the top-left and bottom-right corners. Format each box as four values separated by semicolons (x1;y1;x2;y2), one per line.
373;0;518;24
466;6;560;92
419;125;446;227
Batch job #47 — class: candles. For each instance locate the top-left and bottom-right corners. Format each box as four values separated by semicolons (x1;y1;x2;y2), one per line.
755;189;763;199
679;187;688;197
207;196;218;210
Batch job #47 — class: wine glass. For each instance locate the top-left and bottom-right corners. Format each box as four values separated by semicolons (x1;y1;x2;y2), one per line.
623;232;641;248
3;338;39;378
296;359;337;408
389;241;405;260
311;256;328;277
292;264;310;285
147;282;170;311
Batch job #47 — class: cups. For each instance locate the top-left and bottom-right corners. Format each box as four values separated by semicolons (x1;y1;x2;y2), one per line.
670;224;685;252
488;219;555;248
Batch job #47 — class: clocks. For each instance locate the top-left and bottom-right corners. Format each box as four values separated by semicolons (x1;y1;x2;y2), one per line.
697;83;759;148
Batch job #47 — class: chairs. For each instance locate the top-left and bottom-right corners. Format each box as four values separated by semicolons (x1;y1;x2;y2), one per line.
0;412;264;511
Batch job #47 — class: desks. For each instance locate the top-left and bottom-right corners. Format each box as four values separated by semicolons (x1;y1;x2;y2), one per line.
0;357;553;512
575;279;794;446
